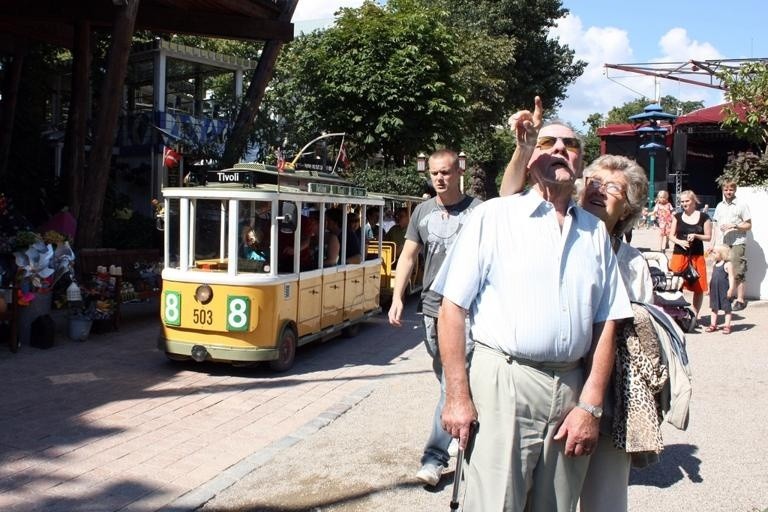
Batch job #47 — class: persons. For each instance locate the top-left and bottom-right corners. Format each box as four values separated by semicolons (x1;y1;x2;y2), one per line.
647;190;674;253
706;181;752;310
705;245;736;334
499;96;655;512
668;190;712;322
238;204;410;274
388;149;485;486
429;118;635;512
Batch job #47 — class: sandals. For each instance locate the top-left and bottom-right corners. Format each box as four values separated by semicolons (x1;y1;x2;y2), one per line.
722;325;733;335
705;324;717;334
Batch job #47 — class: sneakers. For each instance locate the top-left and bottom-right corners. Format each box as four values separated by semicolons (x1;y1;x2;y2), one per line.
733;298;748;310
415;462;444;487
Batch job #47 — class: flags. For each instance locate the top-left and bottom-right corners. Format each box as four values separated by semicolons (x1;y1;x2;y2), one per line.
338;144;350;169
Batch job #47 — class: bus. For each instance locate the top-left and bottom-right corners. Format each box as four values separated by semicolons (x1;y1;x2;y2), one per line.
153;130;430;380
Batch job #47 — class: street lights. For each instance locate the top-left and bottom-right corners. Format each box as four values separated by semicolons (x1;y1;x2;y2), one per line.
628;103;677;209
456;150;468;194
413;148;428;179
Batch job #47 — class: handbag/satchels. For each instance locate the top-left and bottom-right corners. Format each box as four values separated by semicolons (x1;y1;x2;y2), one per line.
679;262;700;287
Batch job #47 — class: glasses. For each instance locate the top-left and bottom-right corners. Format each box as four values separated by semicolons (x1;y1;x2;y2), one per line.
535;134;582;152
585;175;631;207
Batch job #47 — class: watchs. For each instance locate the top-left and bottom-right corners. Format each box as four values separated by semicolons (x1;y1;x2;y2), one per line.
576;400;604;419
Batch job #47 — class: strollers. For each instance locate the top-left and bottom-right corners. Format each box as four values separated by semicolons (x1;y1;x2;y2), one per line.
634;244;699;333
